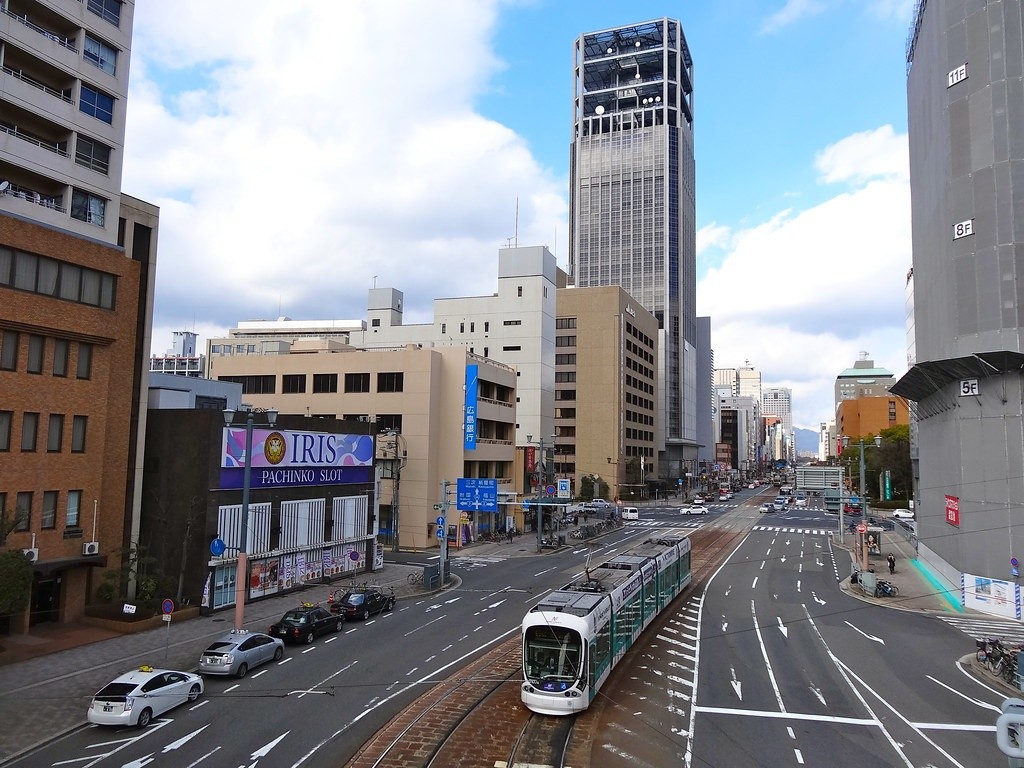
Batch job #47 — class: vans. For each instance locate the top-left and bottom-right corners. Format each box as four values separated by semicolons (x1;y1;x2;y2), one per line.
621;506;639;521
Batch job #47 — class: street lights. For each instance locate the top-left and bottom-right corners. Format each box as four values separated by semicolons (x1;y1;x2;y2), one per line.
841;433;882;573
525;433;558;554
220;405;280;630
606;458;630;520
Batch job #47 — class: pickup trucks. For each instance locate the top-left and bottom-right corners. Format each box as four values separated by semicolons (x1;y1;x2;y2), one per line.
575;502;599;514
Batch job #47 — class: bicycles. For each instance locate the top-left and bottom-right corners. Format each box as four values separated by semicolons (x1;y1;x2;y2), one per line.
975;633;1024;684
333;581;384;601
535;515;623;549
478;529;509;543
875;577;899;598
406;569;425;585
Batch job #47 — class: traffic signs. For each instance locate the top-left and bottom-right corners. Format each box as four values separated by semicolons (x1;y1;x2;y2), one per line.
455;477;498;514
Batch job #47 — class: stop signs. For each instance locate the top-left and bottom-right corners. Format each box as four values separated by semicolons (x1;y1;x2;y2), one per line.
857;523;867;534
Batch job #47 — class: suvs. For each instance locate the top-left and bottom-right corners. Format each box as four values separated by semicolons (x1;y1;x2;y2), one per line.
591;498;611;509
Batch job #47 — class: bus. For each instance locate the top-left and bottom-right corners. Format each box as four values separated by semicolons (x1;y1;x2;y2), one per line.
519;532;694;717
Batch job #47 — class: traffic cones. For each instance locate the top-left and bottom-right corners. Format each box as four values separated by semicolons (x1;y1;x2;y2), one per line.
327;593;336;604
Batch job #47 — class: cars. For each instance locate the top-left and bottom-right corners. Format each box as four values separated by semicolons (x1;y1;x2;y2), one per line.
892;508;914;519
197;629;285;680
85;665;205;729
329;590;394;621
679;504;709;515
693;470;863;518
269;603;346;646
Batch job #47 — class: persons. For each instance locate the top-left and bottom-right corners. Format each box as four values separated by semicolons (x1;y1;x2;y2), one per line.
868;535;881;554
509;528;515;544
887;553;896;575
582;512;588;522
574;514;579;526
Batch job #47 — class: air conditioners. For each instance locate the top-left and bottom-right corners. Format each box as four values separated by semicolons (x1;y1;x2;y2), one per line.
82;542;98;555
22;548;38;561
